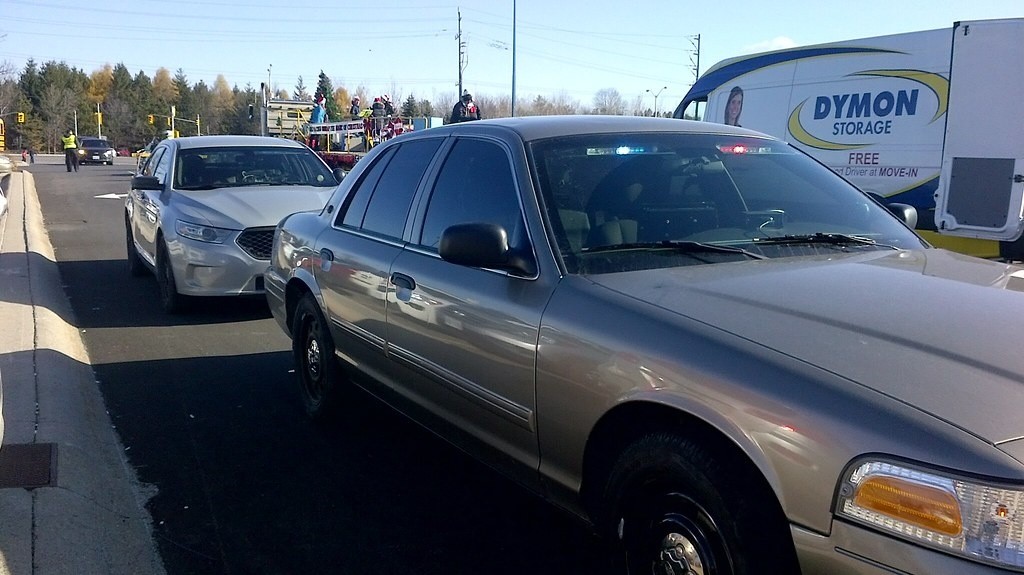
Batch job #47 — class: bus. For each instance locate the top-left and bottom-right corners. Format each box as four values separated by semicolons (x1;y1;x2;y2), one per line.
0;118;5;152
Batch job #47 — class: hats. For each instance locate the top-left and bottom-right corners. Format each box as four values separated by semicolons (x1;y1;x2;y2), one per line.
374;98;380;103
351;95;361;101
381;95;389;101
464;94;472;101
317;97;326;104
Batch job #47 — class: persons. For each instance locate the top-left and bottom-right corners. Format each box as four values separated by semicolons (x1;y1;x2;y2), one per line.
350;96;361;121
163;129;174;140
450;89;481;124
373;94;395;130
724;86;743;127
61;129;81;172
22;147;27;162
309;91;326;150
29;146;37;163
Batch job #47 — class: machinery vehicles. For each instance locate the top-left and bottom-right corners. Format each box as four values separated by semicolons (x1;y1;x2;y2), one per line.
266;98;444;181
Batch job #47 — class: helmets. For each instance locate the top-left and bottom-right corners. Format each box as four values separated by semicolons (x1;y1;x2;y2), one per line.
165;130;172;136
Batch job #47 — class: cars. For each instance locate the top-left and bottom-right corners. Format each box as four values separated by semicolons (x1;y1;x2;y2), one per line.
122;134;348;305
263;111;1024;575
115;148;130;156
77;135;113;166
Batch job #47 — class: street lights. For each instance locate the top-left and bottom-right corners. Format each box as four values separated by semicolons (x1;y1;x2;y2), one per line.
646;85;667;118
266;63;274;101
259;83;266;109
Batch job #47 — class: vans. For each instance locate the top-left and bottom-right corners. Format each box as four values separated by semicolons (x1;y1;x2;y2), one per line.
671;20;1023;267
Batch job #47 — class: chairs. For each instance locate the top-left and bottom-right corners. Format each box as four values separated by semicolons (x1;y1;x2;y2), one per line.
505;162;592;254
179;155;208;189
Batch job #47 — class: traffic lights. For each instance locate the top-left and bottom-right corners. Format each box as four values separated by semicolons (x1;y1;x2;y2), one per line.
248;104;254;122
147;114;154;125
196;120;198;125
18;113;24;123
